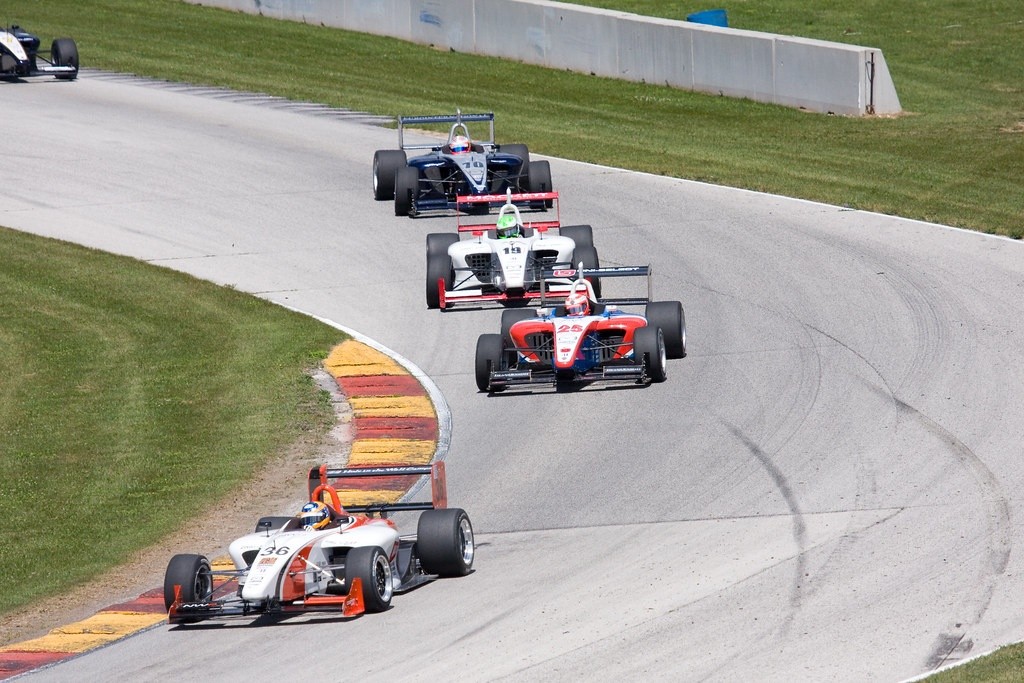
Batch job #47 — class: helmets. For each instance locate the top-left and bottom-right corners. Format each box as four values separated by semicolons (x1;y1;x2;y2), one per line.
449;136;471;154
496;215;520;239
300;501;330;530
565;294;590;317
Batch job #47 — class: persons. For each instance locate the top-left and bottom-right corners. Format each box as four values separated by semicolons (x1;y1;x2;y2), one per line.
495;215;523;238
565;293;591;318
299;501;332;531
449;135;470;154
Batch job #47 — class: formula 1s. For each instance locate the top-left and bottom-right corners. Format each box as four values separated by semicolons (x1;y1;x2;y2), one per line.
421;184;603;309
370;107;556;215
159;460;476;623
0;21;82;82
472;262;689;396
